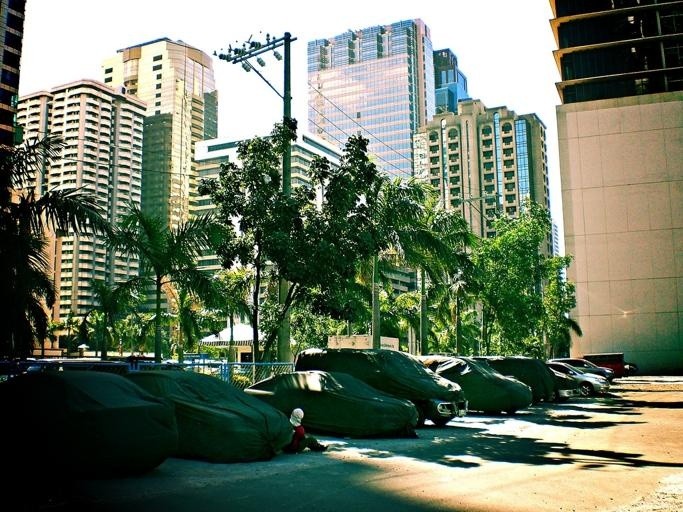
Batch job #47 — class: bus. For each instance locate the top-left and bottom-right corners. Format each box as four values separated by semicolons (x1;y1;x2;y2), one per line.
182;353;209;363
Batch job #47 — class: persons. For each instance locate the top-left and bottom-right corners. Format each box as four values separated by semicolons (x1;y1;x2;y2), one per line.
281;408;326;456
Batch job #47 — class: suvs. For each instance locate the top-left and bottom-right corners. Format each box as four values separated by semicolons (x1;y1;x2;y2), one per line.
293;347;470;428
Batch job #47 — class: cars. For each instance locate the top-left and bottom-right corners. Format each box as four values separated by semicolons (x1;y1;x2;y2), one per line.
0;356;155;377
547;352;638;402
